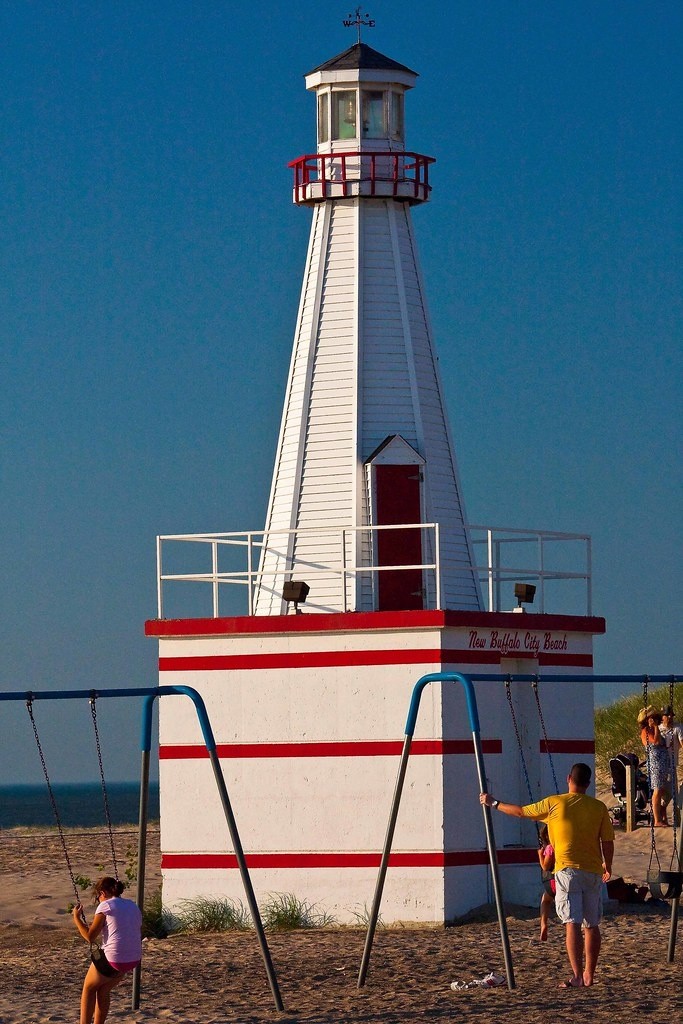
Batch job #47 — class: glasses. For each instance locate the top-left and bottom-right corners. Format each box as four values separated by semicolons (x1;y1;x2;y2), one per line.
95;894;101;900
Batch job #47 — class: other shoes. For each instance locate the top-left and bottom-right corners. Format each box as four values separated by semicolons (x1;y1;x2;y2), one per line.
654;823;668;827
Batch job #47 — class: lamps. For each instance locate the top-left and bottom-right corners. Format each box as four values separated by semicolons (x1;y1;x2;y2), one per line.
512;583;536;613
282;580;310;615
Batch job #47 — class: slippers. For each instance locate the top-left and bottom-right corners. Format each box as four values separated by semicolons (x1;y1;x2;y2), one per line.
556;979;572;988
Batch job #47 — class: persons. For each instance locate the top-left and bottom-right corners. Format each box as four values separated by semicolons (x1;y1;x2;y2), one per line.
479;763;615;988
538;825;556;941
638;705;669;827
658;706;683;828
73;877;142;1024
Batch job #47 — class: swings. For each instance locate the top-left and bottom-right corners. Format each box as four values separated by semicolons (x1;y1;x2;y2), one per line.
506;687;560;897
26;701;129;979
643;687;683;899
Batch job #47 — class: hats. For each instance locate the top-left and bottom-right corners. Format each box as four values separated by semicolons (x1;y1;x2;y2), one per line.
660;705;675;717
638;704;661;724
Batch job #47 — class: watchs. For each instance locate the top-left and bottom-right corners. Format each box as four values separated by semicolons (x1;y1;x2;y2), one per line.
492;800;499;809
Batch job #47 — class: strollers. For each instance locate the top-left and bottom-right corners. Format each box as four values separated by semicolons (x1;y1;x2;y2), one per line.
607;752;652;827
